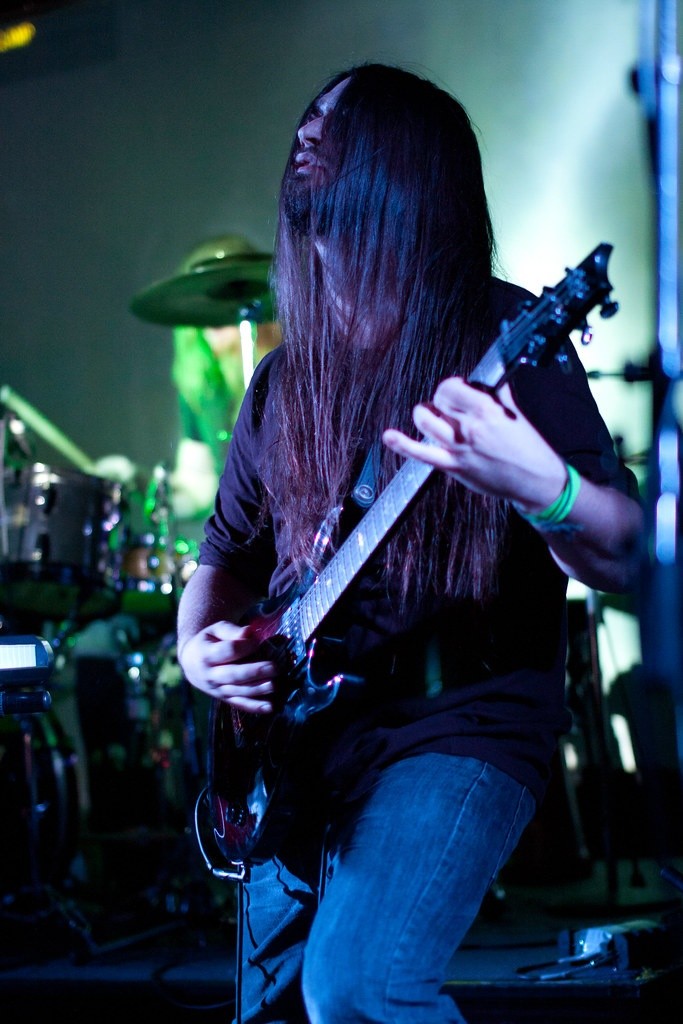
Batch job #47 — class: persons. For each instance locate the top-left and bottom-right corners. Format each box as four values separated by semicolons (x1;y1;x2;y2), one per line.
175;61;655;1024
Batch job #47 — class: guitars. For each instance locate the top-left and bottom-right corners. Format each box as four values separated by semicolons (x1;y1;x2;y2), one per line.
188;238;620;870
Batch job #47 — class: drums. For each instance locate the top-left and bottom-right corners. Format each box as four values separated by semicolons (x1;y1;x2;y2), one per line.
39;603;158;756
105;468;180;612
2;459;131;621
149;638;209;795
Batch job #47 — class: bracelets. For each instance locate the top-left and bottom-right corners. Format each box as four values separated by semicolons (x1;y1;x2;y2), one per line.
521;462;586;537
